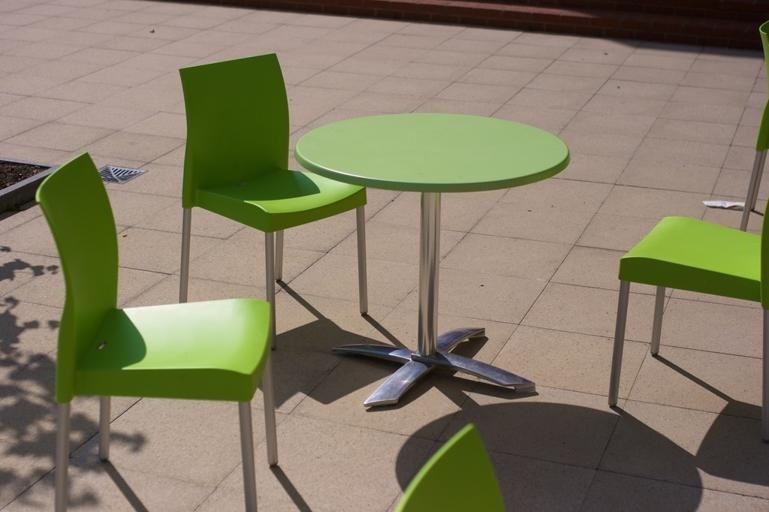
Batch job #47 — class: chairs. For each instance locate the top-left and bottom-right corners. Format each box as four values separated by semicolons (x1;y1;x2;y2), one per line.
740;19;769;232
607;197;769;444
396;424;506;511
36;151;278;512
178;52;367;350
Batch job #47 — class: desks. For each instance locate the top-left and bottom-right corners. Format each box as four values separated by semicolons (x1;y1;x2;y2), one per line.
295;111;571;404
